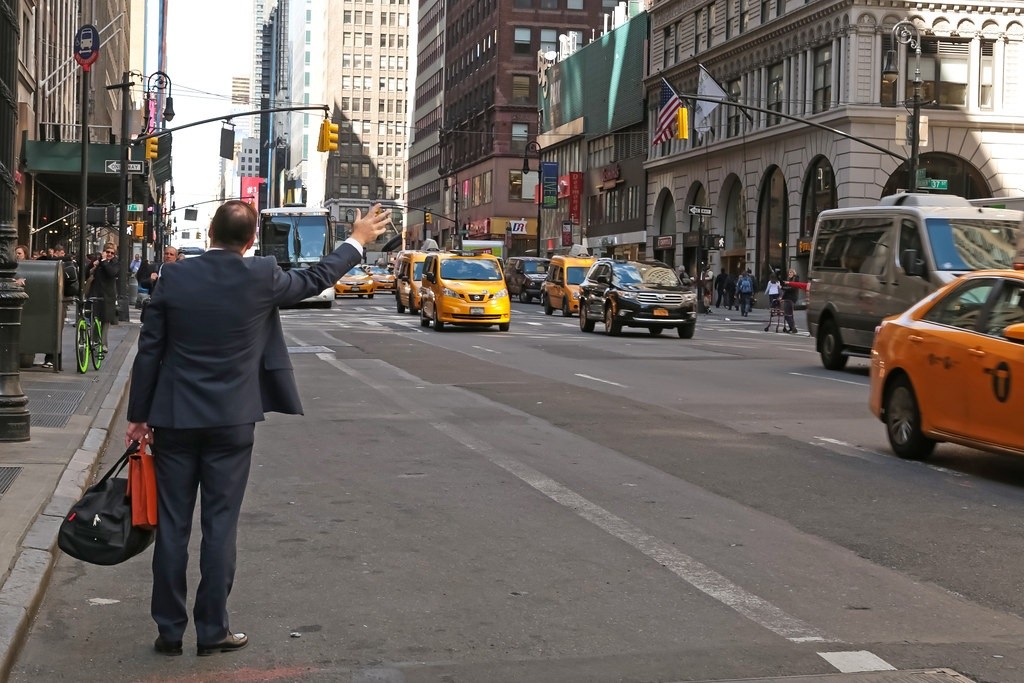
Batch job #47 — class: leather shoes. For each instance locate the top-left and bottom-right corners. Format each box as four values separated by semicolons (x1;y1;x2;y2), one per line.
197;631;249;656
154;637;182;656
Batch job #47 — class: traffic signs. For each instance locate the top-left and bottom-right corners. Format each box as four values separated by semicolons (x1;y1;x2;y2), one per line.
103;159;145;175
687;204;713;217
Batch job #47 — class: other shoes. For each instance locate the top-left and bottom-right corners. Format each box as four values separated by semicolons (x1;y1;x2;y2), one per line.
41;361;53;368
741;312;744;316
728;307;732;310
788;327;798;333
736;306;739;311
744;312;748;317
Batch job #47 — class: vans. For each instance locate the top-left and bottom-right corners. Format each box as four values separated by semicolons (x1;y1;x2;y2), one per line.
543;243;598;317
419;248;511;332
806;191;1019;371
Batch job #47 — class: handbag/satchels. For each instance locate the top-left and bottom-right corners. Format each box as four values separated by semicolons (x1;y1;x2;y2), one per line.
125;439;158;530
140;297;151;323
55;439;155;567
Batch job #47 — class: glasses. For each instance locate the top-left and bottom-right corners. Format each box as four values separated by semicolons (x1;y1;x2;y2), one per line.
106;251;114;255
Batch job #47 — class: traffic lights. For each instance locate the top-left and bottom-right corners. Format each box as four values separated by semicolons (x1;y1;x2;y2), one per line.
322;119;340;151
146;133;159;160
425;212;432;224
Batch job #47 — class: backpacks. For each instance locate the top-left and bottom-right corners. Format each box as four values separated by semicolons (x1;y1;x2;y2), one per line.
740;277;751;293
61;262;80;296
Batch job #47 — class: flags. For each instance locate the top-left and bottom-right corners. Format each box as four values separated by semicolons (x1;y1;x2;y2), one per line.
653;76;685;146
694;64;727;135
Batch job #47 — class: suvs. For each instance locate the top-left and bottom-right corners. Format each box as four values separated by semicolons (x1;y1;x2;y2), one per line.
502;256;551;304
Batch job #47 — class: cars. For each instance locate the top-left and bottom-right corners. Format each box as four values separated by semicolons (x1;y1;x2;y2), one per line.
333;249;460;316
577;256;699;340
866;263;1024;460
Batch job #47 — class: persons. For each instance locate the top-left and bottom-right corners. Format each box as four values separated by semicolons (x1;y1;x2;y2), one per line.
15;242;187;354
678;265;808;333
125;200;393;656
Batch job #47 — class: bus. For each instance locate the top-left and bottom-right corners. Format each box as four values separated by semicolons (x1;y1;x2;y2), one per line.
177;246;202;259
254;202;336;309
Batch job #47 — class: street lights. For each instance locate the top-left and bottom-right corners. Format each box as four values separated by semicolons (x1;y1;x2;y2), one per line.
881;16;937;193
443;168;459;235
135;70;176;308
521;140;543;257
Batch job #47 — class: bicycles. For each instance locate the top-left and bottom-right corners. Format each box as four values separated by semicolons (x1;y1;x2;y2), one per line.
61;295;106;375
